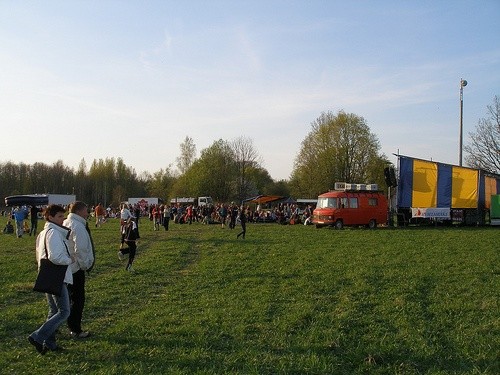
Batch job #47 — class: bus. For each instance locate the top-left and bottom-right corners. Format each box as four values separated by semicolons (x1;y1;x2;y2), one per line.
313;182;388;229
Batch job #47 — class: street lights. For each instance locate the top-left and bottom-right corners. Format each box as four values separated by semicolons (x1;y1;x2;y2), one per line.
460;81;467;166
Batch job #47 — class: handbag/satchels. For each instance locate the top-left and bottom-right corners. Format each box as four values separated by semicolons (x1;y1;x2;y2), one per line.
32;258;68;295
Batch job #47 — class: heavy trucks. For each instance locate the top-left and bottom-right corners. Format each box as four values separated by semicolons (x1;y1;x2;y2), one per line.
171;196;214;208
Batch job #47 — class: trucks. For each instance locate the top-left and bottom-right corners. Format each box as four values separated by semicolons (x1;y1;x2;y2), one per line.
119;198;160;211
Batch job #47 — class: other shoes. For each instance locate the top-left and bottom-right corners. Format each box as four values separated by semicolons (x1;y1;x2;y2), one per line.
125;265;136;272
27;336;44;355
43;345;68;352
117;251;125;261
70;330;91;338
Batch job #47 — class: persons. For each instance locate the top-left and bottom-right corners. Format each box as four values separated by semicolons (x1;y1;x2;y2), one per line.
0;201;319;239
63;200;96;340
25;203;79;354
236;206;247;240
118;217;141;272
